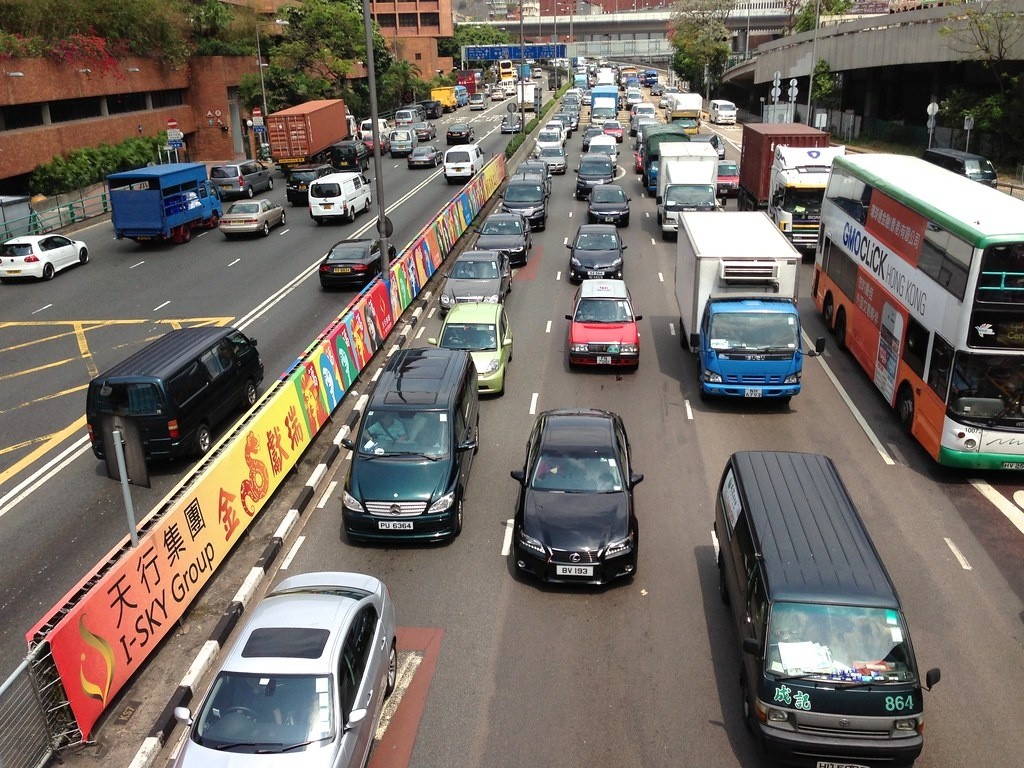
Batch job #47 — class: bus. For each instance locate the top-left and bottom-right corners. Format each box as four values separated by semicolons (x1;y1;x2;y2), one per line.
809;153;1024;471
457;61;512;94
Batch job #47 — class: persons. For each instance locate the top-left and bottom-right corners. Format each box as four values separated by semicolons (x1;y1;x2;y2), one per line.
577;303;596;316
460;262;475;278
903;6;908;25
536;452;567;481
916;5;922;24
923;4;929;23
930;4;933;21
449;330;466;342
711;317;737;339
486;223;499;234
945;352;993;398
910;7;914;11
896;8;901;13
938;2;943;21
890;9;895;26
236;677;282;725
363;414;410;442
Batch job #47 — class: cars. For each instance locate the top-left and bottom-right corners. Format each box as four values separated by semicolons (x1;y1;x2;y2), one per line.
713;137;740;198
428;86;590;395
0;234;89;280
633;143;644;173
510;408;644;593
564;186;642;370
174;572;396;768
581;120;624;150
219;198;396;290
500;115;522;134
363;121;475;169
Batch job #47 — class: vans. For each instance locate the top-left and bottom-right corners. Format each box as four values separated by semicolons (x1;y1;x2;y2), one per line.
340;348;479;544
86;325;264;461
394;98;442;127
714;450;941;768
622;76;658;148
923;147;998;189
470;78;517;110
573;133;620;200
210;118;417;224
443;144;486;183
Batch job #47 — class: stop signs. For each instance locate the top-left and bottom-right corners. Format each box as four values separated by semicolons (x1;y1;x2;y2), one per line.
167;119;177;128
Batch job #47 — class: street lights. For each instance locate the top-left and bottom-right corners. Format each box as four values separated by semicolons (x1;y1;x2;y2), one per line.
256;19;290;119
526;2;586;94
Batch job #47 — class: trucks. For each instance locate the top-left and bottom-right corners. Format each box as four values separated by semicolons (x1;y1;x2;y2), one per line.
642;93;825;402
264;98;358;175
107;161;223;243
516;56;637;123
